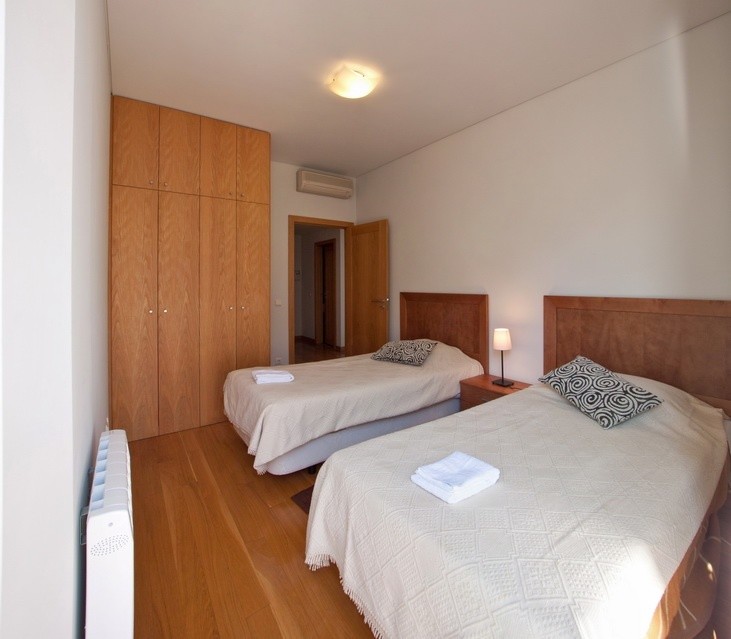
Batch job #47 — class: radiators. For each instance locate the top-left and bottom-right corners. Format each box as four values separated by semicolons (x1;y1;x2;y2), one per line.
81;424;140;639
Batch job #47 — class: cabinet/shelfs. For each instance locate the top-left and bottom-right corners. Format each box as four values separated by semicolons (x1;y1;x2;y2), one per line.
104;91;277;454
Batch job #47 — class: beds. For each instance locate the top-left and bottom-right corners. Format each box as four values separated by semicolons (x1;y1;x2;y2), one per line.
202;291;492;478
302;294;731;639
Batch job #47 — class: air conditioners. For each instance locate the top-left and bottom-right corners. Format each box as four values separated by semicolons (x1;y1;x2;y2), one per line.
293;166;358;204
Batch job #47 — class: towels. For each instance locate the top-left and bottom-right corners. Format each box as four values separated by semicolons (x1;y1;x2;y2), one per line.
411;450;499;505
250;370;294;383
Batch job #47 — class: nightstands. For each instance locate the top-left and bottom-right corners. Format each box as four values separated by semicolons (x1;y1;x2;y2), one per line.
457;372;530;423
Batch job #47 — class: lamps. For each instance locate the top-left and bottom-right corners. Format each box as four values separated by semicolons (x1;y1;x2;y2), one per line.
489;327;516;392
325;63;379;104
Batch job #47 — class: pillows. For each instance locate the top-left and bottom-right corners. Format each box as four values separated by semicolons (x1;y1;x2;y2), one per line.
533;354;666;430
369;336;438;367
